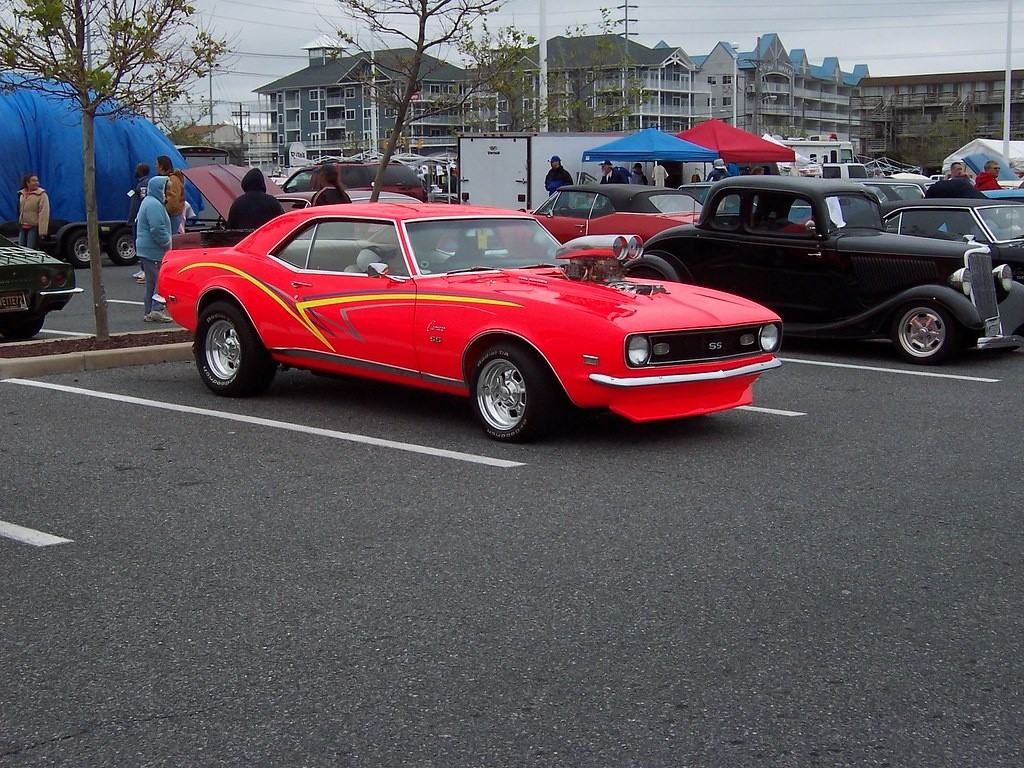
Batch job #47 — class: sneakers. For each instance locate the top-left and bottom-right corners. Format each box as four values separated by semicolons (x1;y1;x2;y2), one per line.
133;271;145;278
145;309;172;324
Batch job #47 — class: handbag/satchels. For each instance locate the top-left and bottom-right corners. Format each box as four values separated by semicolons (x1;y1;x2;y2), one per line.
185;201;196;219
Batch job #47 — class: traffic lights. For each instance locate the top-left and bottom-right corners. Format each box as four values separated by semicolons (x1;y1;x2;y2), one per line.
400;137;405;147
419;138;424;149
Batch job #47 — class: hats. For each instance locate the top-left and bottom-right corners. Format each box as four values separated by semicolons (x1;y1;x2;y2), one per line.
551;156;560;164
599;160;612;165
633;163;641;168
314;165;338;176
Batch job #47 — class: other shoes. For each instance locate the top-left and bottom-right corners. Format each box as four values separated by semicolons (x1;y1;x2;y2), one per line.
136;278;145;283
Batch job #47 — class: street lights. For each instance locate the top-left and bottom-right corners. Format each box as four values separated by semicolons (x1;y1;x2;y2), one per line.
759;94;777;140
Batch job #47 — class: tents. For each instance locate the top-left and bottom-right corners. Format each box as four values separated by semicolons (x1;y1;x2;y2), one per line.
576;127;719;185
762;134;810;165
677;118;796;167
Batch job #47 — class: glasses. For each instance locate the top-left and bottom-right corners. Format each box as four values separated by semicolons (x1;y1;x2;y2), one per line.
994;167;1001;170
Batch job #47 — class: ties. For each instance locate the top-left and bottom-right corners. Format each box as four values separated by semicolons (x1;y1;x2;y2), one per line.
608;175;610;182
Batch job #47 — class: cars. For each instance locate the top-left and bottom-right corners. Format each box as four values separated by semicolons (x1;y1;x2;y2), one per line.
158;202;784;444
171;165;423;250
281;163;428;203
515;184;703;246
678;134;1024;205
0;234;84;340
843;199;1023;285
620;175;1024;365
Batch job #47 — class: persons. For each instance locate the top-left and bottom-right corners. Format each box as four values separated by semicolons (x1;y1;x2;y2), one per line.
631;163;648;185
873;168;884;178
707;159;731;181
949;162;974;186
316;164;351;205
974;161;1001;190
442;168;457;193
545;156;573;207
226;168;284;229
599;160;626;183
751;165;764;175
132;156;195;322
16;174;50;248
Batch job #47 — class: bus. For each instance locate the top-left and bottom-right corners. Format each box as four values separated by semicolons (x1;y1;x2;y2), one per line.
174;145;231;230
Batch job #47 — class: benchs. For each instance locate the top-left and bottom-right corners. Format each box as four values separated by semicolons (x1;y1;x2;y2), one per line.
279;240;409;276
